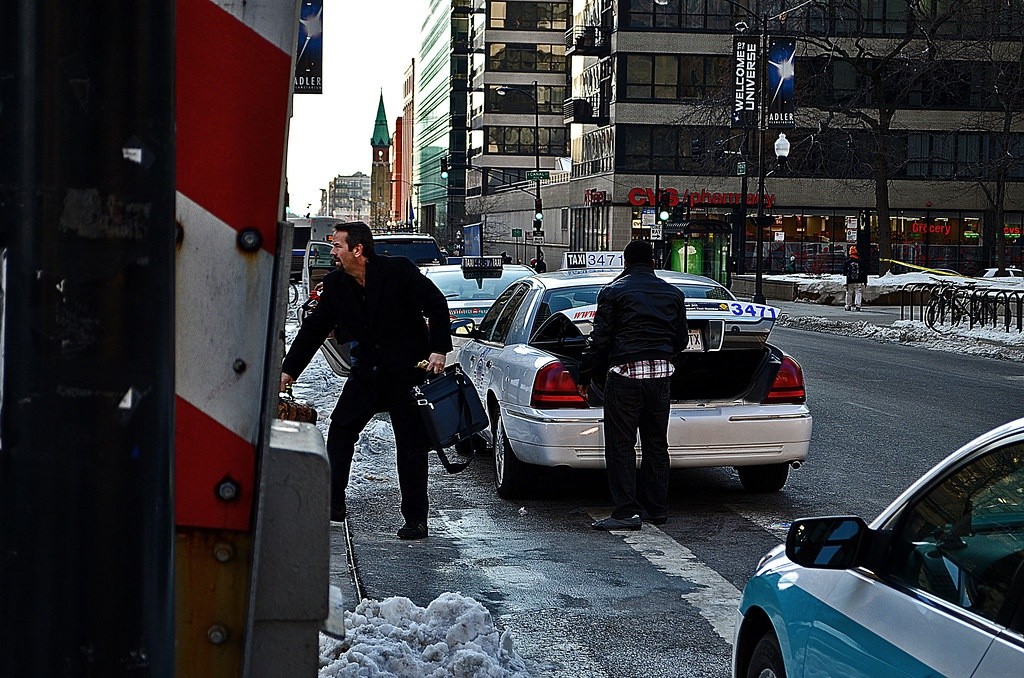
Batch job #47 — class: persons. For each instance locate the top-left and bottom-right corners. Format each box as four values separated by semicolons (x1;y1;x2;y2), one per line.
500;252;547;272
591;239;689;530
842;246;867;311
280;222;453;539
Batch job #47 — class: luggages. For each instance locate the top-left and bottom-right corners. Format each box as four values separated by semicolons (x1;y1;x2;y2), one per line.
277;384;317;424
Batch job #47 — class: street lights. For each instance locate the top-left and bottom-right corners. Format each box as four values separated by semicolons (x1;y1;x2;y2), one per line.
495;80;541;260
389;180;419;233
413;183;453;241
752;132;790;305
349;196;377;233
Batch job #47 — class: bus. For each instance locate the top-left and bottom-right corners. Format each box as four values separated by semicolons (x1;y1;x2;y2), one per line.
286;217;347;281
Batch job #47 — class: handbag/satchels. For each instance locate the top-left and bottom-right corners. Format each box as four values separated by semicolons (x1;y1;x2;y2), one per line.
410;363;490;475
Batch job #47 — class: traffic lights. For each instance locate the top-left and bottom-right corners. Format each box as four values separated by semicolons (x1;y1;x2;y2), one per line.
535;199;543;219
714;140;725;167
675;203;683;223
441;157;448;179
691;138;701;163
659;192;671;221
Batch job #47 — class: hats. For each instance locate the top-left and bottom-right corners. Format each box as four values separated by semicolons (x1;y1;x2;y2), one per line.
849;246;857;254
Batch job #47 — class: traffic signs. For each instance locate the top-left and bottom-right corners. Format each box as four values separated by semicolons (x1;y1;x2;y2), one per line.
651;224;662;240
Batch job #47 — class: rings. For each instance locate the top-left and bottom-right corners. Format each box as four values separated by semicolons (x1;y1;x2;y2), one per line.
439;368;443;371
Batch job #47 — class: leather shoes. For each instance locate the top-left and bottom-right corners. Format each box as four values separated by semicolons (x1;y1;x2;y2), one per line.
643;514;668;524
591;517;642;530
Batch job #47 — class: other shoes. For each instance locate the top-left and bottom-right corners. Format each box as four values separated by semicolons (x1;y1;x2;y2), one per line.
844;305;851;311
852;306;860;312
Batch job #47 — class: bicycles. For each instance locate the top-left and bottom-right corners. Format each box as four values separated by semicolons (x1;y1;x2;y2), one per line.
924;276;1012;333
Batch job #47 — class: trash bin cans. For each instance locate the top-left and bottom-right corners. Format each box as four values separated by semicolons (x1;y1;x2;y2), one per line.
246;420;332;678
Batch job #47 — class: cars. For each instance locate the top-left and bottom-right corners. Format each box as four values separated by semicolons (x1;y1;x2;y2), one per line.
973;267;1024;278
302;233;447;302
296;256;538;377
448;250;813;500
730;417;1023;677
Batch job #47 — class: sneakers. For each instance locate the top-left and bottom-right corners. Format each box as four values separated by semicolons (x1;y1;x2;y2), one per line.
330;507;346;521
397;521;428;539
